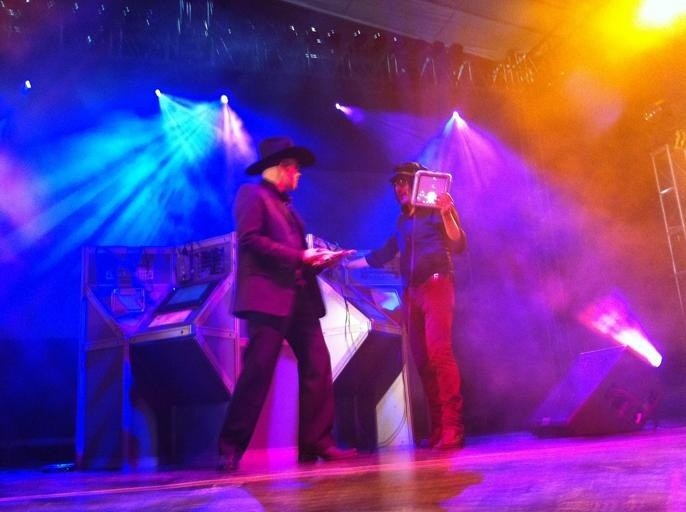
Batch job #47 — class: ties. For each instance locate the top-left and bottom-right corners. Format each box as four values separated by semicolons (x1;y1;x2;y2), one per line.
168;281;213;305
371;288;402;313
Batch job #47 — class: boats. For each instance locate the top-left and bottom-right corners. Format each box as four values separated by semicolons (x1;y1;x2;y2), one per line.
523;344;668;438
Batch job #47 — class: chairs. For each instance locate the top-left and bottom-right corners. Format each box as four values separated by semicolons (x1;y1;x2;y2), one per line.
215;448;241;472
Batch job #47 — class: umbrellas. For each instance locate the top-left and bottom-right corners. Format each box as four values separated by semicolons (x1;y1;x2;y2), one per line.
298;446;359;464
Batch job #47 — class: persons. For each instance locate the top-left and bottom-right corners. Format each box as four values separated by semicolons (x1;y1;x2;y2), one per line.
212;136;357;474
337;162;465;451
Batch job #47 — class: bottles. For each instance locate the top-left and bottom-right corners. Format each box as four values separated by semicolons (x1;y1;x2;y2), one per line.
388;160;429;183
246;136;315;177
